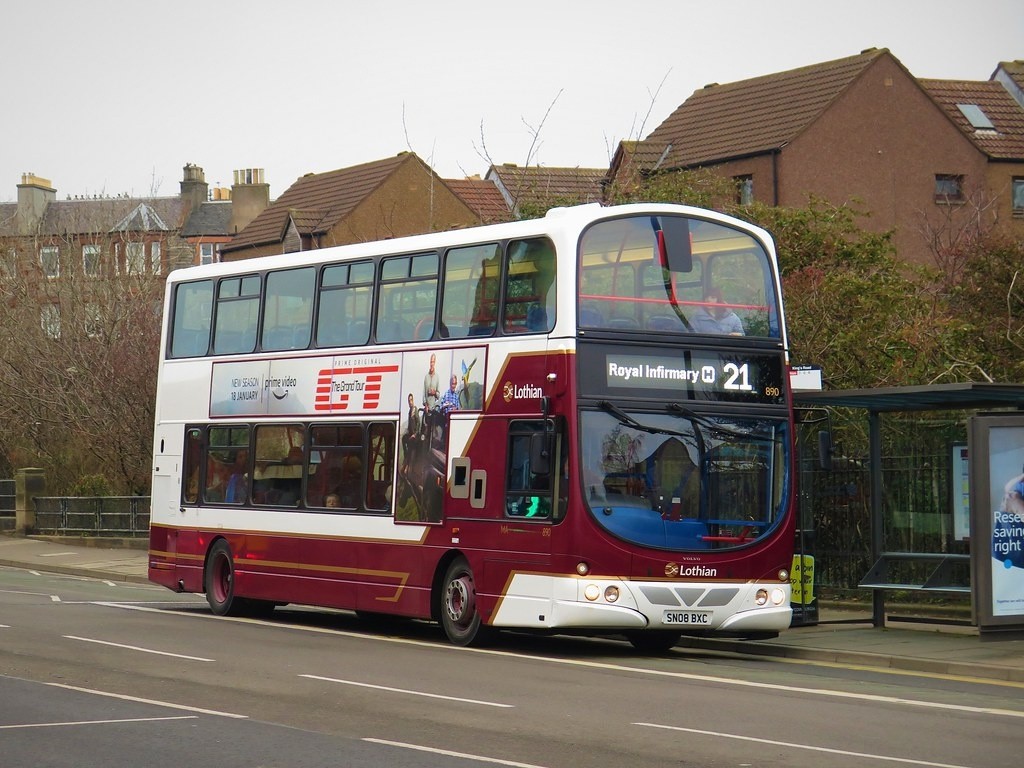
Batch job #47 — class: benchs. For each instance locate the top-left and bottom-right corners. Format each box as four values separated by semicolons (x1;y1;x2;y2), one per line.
855;551;971;628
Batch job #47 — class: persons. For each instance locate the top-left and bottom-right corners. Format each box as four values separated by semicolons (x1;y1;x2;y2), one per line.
310;431;362;507
691;289;745;335
402;354;461;470
188;447;303;502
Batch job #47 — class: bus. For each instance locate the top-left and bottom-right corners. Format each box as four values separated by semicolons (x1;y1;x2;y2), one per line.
145;200;835;658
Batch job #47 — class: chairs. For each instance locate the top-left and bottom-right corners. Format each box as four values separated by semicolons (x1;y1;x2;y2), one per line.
194;315;681;355
